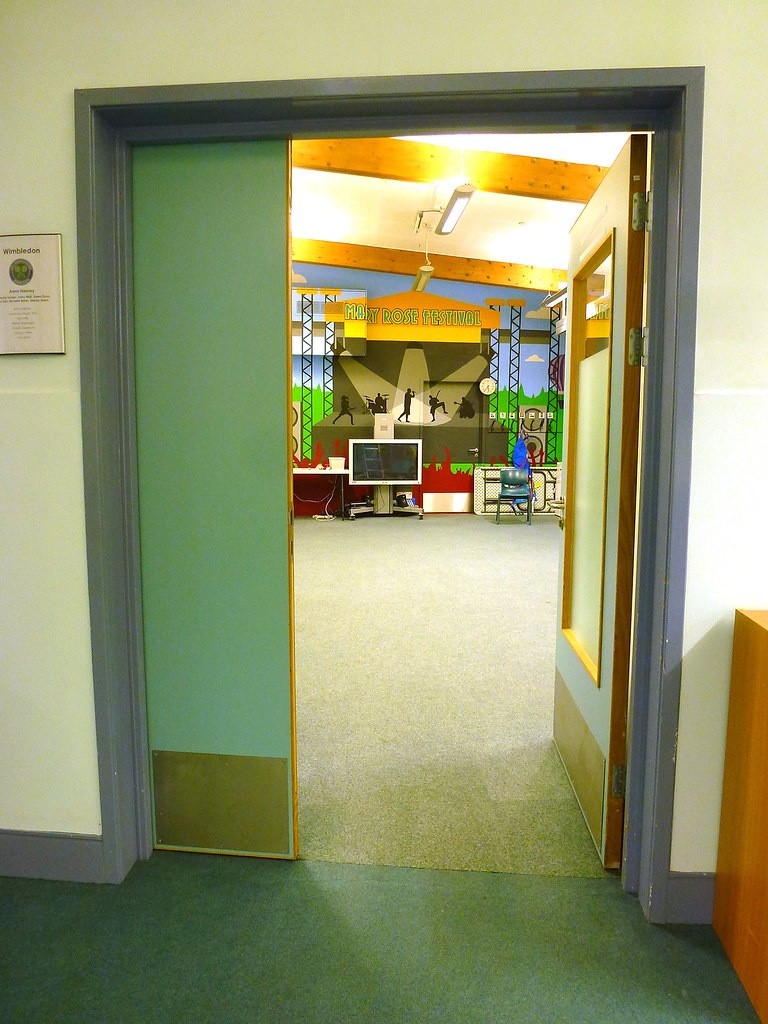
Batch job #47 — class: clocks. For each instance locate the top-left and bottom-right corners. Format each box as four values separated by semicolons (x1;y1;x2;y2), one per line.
479;378;495;395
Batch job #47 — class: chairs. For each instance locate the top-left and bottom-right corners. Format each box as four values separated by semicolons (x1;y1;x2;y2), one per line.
496;470;531;526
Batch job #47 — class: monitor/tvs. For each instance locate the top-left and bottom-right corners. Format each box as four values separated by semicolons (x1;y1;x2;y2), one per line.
348;438;422;486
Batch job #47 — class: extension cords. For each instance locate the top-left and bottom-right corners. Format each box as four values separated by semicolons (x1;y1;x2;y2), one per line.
313;514;334;519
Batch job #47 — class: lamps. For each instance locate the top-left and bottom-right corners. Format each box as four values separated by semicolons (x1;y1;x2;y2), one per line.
415;212;423;234
539;286;567;308
435;184;477;236
412;234;434;292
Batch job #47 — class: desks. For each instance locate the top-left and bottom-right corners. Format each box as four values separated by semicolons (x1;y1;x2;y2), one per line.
293;468;349;521
474;467;558;515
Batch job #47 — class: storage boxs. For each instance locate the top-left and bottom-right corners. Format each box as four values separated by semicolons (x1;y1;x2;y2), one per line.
328;457;346;471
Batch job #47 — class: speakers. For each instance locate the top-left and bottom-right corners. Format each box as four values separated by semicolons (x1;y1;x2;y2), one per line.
519;404;549;464
